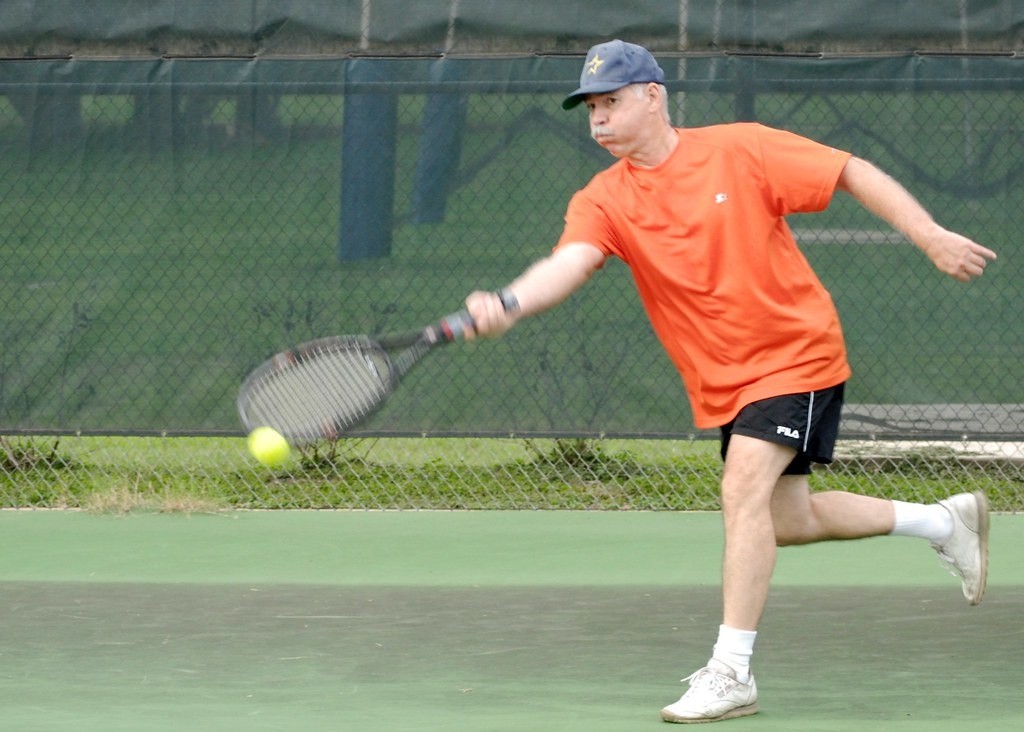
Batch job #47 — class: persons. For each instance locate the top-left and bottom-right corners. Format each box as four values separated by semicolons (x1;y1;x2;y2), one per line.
461;38;999;724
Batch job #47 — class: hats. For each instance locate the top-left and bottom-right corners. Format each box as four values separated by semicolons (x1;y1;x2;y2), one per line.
562;38;665;109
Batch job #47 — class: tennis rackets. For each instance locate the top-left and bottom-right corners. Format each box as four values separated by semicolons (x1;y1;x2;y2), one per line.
233;290;520;447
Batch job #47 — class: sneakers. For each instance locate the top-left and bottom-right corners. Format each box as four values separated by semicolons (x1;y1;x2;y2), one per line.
660;657;760;723
929;492;990;605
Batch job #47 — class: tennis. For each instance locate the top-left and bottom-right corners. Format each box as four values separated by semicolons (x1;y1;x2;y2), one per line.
249;425;290;466
